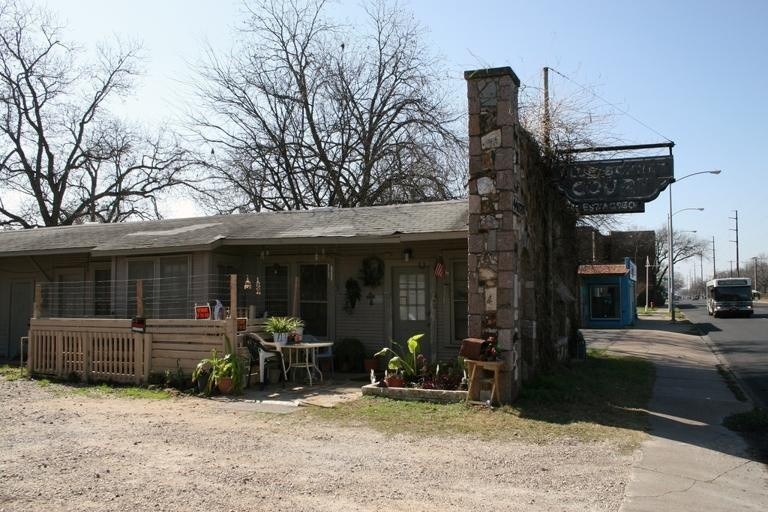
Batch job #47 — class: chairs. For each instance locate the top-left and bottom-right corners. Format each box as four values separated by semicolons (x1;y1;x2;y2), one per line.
245;331;289;389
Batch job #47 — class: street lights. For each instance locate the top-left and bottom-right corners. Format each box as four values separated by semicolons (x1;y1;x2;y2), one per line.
667;169;721;324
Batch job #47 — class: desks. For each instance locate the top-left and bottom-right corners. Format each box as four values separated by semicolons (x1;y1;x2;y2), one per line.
280;341;335;387
463;358;505;408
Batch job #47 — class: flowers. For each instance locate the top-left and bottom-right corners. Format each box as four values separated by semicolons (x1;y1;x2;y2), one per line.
485;336;497;357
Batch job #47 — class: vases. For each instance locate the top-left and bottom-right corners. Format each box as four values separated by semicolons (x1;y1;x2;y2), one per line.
487;356;494;361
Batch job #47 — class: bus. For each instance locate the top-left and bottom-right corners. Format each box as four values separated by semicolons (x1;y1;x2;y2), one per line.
705;278;754;319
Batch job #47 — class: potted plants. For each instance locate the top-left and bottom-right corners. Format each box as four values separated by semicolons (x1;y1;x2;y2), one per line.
191;335;244;396
265;316;306;345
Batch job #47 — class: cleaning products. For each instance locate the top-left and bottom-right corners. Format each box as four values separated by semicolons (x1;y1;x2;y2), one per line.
213;298;224;321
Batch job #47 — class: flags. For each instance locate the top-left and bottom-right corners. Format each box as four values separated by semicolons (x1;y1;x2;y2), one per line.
433;256;445;277
